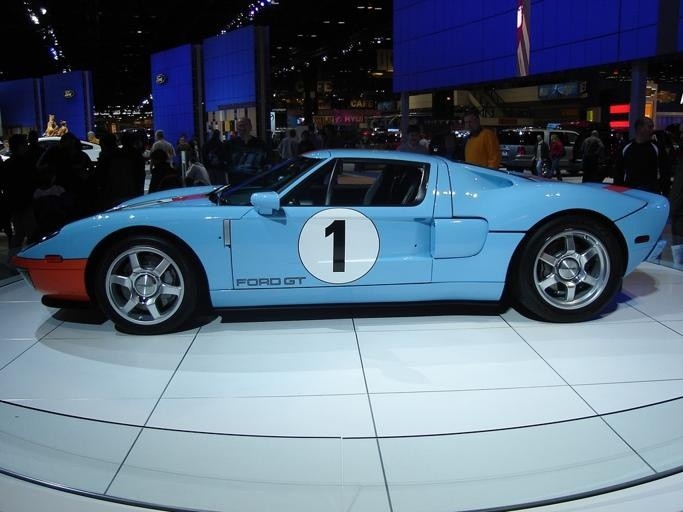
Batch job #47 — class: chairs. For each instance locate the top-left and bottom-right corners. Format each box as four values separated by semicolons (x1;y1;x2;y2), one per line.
362;165;419;205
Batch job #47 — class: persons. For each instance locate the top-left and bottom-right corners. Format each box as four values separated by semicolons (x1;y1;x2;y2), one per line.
534;134;564;181
149;117;273;192
1;129;146;252
277;124;344;161
574;129;605;183
617;117;681;259
368;113;500;170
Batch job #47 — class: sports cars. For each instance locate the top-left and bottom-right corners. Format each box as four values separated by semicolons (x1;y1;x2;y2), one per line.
9;147;671;323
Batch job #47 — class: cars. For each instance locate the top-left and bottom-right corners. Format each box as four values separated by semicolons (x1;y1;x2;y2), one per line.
0;135;101;161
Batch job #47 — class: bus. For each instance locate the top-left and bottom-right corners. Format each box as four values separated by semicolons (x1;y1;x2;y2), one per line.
362;115;392;144
386;105;481;143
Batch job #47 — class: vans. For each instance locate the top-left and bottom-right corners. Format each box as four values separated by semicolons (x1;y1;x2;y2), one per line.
500;129;586;175
